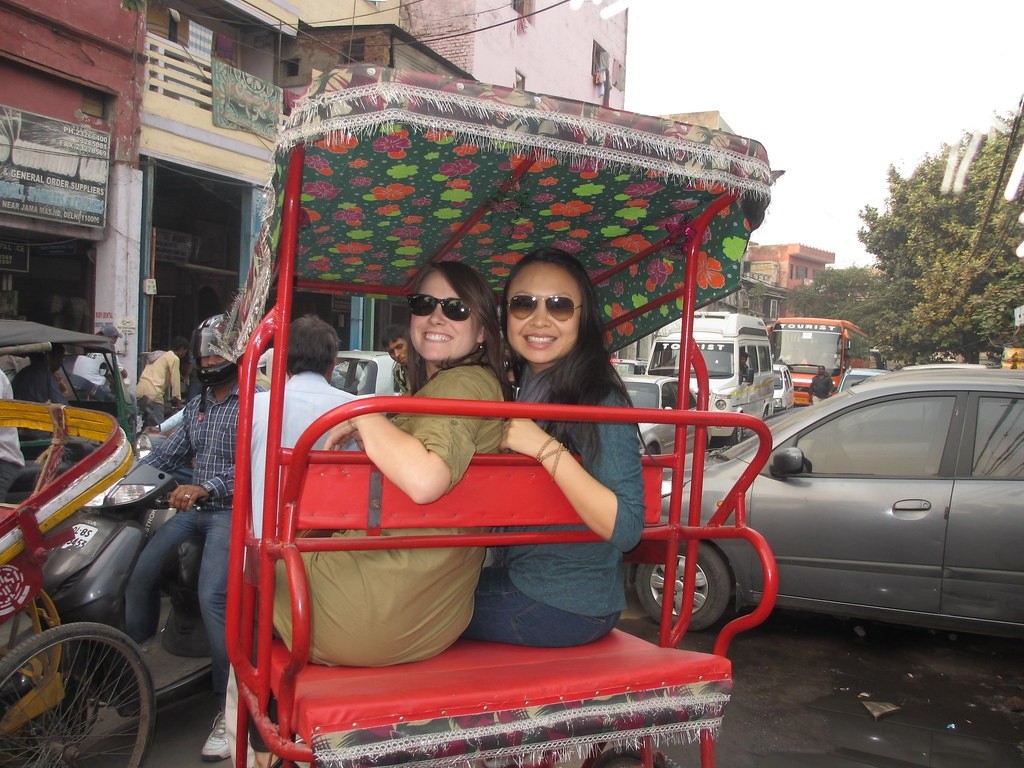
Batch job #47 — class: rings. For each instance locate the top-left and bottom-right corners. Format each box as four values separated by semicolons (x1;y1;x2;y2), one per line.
184;493;190;498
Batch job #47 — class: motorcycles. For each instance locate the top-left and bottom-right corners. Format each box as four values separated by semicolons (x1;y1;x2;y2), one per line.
2;460;228;768
1;317;136;481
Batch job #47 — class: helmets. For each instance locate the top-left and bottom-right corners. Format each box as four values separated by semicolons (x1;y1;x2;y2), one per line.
189;313;239;387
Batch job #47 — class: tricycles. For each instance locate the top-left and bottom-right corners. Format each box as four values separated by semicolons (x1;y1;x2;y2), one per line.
224;61;783;767
0;398;159;768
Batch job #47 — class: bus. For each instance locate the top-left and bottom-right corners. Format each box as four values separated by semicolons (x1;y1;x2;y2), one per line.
642;314;873;446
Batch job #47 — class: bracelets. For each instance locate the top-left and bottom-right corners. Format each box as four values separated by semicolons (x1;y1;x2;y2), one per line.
535;437;570;482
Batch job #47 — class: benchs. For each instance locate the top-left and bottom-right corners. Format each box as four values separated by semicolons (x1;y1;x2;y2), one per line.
259;394;780;745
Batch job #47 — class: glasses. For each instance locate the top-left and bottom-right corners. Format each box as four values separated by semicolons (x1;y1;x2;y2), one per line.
405;294;475;321
506;295;585;322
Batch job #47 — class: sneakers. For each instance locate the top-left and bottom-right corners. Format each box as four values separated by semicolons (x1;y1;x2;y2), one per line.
201;712;230;761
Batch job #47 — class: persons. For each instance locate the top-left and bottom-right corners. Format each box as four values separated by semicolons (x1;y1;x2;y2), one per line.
808;365;836;405
123;313;266;762
251;313;370;540
0;322;413;504
271;260;509;667
738;351;748;384
458;247;647;646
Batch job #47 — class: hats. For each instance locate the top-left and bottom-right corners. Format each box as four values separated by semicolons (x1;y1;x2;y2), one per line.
100;325;123;338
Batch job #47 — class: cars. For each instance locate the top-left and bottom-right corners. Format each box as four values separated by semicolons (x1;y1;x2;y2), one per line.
626;363;1023;640
140;349;709;458
